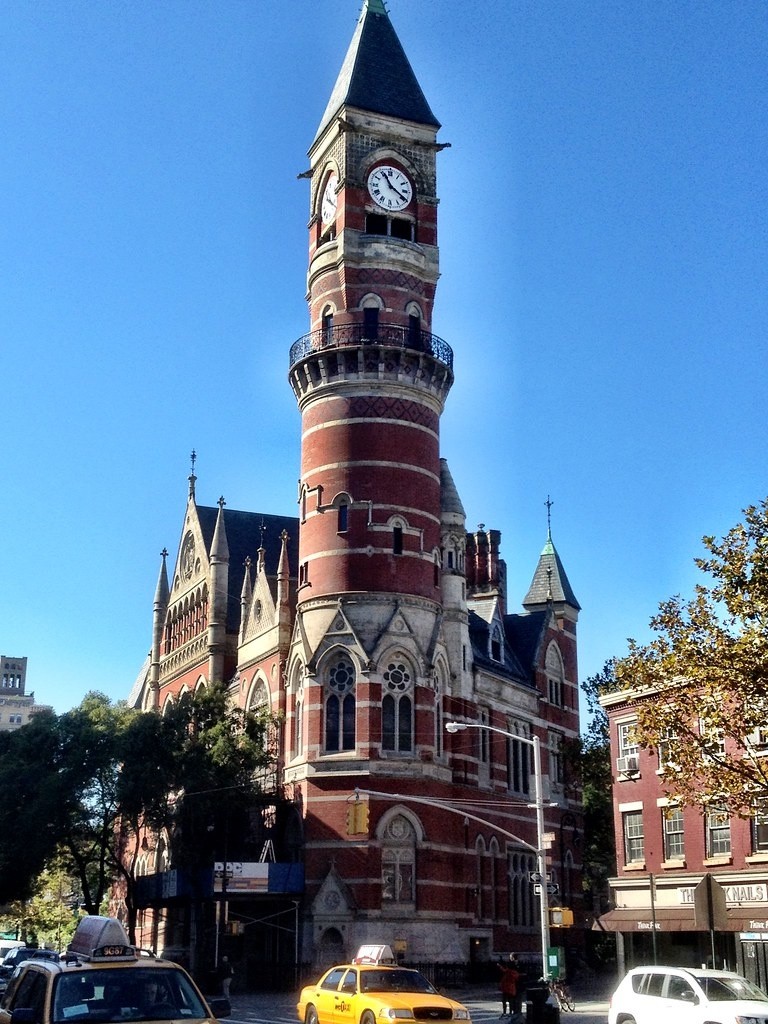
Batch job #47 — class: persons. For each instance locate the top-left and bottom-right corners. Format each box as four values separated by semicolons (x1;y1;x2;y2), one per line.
219;956;235;999
497;952;528;1016
137;977;167;1009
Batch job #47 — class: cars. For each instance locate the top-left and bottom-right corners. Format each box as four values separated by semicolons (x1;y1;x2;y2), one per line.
296;945;473;1024
0;939;60;999
0;914;231;1024
607;964;768;1024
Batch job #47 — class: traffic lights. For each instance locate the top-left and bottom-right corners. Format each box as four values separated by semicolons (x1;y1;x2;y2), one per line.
355;801;371;834
346;805;354;837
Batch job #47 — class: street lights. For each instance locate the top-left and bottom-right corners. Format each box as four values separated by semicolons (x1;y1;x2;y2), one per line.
446;721;550;1007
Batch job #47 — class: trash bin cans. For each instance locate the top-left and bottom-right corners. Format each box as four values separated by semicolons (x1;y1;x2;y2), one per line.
523;980;553;1024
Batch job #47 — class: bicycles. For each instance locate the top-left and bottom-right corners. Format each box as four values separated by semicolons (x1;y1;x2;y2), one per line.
545;970;576;1012
